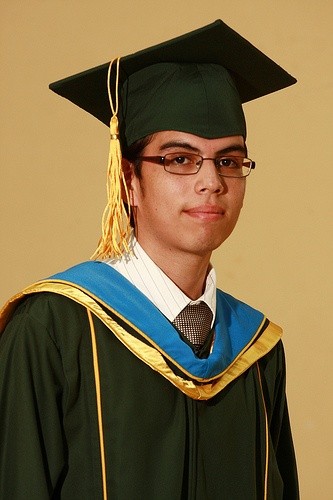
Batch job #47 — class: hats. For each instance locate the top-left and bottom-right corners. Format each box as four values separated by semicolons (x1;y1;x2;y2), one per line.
48;18;297;260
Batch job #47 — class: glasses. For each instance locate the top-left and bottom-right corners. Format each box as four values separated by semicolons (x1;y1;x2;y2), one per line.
130;152;256;179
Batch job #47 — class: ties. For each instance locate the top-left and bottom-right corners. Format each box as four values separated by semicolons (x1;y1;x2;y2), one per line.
172;302;213;351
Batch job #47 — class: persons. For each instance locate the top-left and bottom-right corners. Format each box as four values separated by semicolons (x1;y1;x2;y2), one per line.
0;20;301;500
